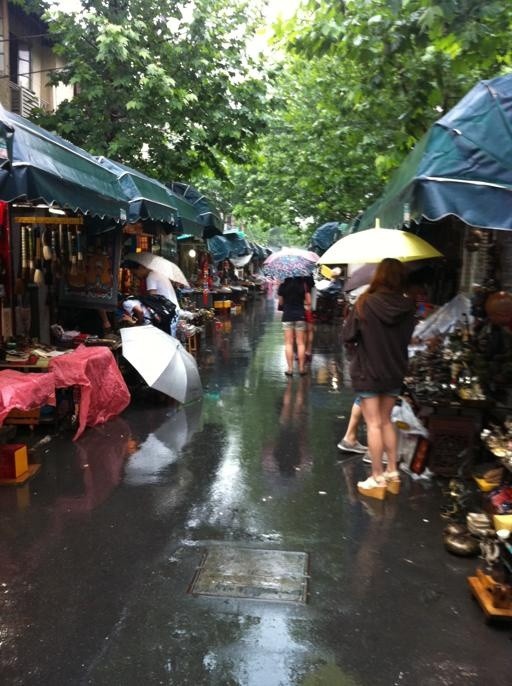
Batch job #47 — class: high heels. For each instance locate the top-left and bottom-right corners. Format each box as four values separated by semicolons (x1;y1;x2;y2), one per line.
357;470;401;500
337;438;368;454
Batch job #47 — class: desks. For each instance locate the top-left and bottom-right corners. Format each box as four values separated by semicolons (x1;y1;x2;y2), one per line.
1;340;122;432
410;402;477;477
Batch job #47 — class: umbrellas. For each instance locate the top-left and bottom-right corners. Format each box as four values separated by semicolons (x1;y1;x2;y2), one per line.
118;322;206;407
315;218;445;266
122;399;205;489
123;252;190;288
261;254;319;283
262;247;321;264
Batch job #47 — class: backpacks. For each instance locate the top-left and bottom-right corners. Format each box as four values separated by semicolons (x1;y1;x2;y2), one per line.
120;294;177;324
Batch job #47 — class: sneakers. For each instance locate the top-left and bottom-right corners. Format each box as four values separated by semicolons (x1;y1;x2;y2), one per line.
361;451;393;464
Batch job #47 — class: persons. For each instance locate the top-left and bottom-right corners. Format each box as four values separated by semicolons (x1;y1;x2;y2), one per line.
111;292;173;338
313;267;341;322
277;278;312;376
342;259;419;503
272;377;307;480
336;260;403;466
292;276;315;364
126;262;181;340
330;453;379;557
316;358;346;422
320;495;401;675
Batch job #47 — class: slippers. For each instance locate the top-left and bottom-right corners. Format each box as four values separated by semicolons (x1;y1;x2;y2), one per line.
286;370;307;376
295;351;313;361
